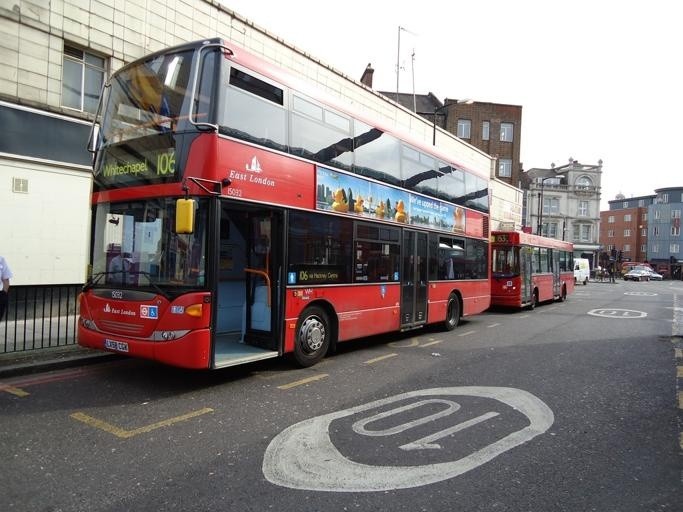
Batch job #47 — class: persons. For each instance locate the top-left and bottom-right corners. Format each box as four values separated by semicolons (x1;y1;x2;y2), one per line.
0;256;11;322
107;252;133;286
606;259;615;283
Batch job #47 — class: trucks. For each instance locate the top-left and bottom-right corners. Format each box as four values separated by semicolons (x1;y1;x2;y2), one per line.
619;262;663;281
558;258;591;286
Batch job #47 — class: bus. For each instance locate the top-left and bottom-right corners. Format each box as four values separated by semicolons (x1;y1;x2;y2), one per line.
75;36;497;374
489;228;574;311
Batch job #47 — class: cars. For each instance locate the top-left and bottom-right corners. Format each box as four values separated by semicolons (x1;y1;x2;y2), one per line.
598;265;609;276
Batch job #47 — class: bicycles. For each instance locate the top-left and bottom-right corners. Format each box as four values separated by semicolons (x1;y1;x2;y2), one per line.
594;272;611;282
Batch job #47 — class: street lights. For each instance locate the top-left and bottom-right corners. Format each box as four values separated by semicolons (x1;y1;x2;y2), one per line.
536;174;568;235
